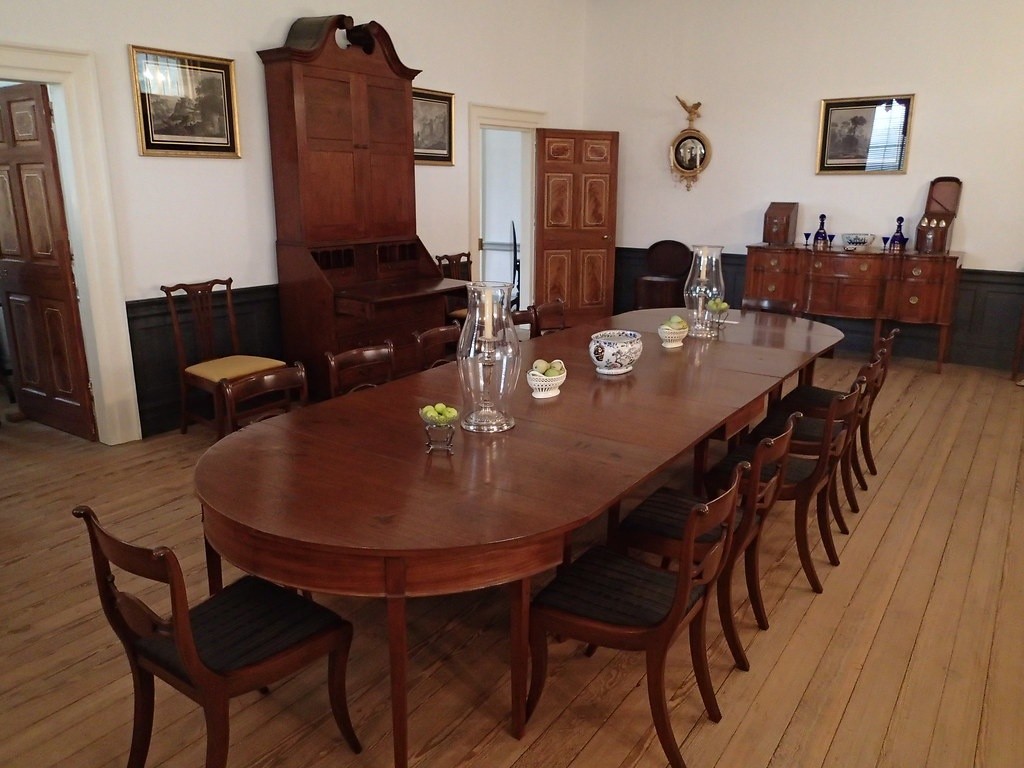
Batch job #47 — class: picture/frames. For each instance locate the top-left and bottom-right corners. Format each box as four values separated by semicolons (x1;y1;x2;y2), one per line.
125;42;242;158
411;85;456;167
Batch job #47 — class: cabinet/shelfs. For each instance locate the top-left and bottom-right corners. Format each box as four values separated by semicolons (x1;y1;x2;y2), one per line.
258;13;468;399
745;240;958;374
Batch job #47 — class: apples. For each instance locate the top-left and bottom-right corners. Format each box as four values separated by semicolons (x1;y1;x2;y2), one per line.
532;359;565;377
422;402;457;424
663;315;687;330
708;298;728;312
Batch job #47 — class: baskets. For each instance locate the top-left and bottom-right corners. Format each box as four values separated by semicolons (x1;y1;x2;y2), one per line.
527;359;567;399
658;326;687;348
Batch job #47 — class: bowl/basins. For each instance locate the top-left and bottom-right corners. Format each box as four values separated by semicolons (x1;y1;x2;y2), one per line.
842;233;875;252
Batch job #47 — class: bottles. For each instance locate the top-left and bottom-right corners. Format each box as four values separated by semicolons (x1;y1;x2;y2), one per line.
683;244;725;340
813;214;829;251
589;329;643;375
889;217;905;256
456;281;522;434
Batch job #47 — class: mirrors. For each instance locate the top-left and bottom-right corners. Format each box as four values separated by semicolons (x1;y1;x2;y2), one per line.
814;91;917;175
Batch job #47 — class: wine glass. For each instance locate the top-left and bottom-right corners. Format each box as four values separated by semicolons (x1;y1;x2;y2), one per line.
828;234;836;248
803;233;811;246
904;238;909;252
881;237;889;251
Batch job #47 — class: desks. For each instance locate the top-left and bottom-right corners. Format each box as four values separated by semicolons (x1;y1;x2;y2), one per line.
191;303;843;766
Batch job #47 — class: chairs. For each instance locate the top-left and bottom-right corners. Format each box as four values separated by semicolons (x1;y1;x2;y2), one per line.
519;323;901;765
435;249;472;328
214;295;567;600
70;503;361;767
159;276;286;438
